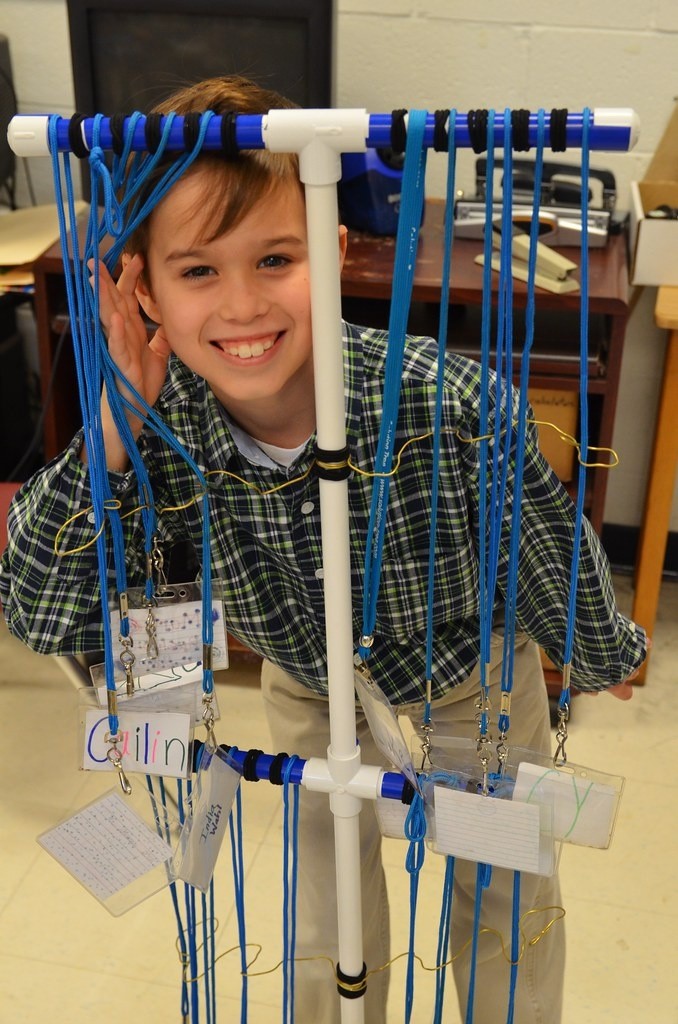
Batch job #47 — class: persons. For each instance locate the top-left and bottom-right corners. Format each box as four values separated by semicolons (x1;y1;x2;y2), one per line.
0;74;651;1024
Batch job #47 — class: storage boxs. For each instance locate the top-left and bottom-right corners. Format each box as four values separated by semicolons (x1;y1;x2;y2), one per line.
629;104;678;288
528;388;578;482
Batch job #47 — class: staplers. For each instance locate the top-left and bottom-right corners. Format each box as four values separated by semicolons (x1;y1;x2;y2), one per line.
474;220;583;295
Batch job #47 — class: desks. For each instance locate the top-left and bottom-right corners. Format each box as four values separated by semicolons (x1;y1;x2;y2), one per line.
30;199;630;699
624;284;678;687
